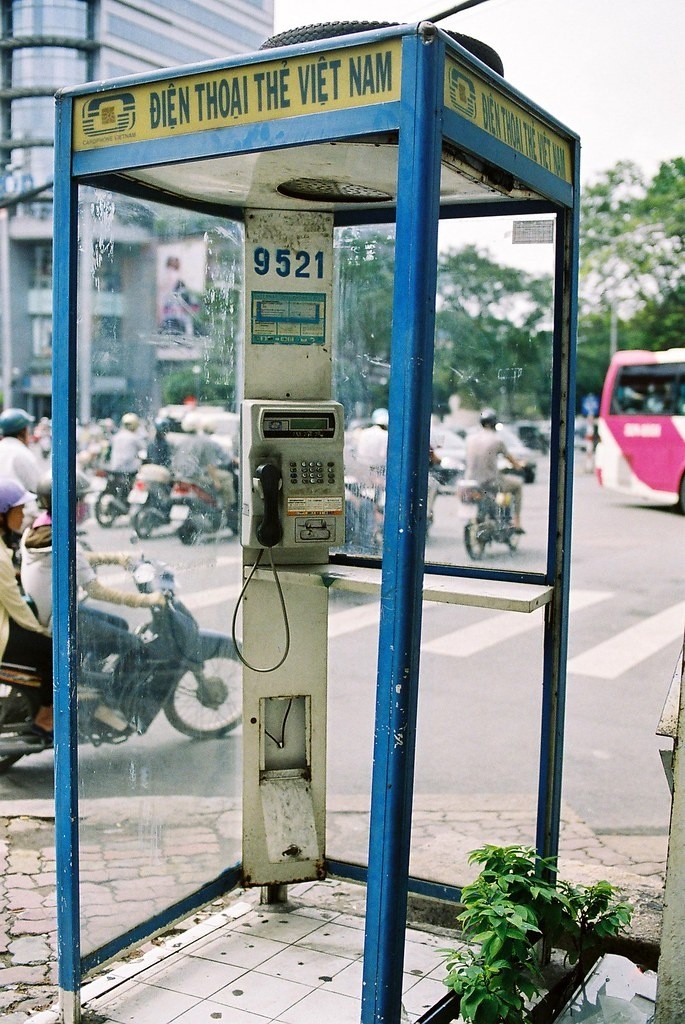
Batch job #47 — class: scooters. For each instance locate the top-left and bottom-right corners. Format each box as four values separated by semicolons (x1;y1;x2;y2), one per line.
33;430;54;460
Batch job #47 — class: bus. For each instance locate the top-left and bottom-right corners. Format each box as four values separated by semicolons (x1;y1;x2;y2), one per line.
596;347;685;512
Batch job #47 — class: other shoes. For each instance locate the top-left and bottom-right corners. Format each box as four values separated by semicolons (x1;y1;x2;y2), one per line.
29;722;53;737
85;714;133;736
227;507;238;534
511;527;524;535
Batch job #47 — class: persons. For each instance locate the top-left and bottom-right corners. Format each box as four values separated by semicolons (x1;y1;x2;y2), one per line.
341;394;478;524
623;384;644;409
35;407;239;513
468;409;526;535
20;472;166;737
160;256;204;335
0;408;53;554
0;479;55;741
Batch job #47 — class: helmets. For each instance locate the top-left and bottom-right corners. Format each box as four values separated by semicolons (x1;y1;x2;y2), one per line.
37;470;95;509
122;413;138;431
0;408;35;435
0;480;38;513
480;408;496;423
372;408;389;429
199;415;218;435
182;412;203;433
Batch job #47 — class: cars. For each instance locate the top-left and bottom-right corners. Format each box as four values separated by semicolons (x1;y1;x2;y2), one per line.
430;423;536;487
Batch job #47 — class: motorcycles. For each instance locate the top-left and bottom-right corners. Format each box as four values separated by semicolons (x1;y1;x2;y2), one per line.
171;460;241;540
130;462;180;537
516;420;554;459
1;556;243;767
338;460;430;559
459;463;533;558
94;455;138;529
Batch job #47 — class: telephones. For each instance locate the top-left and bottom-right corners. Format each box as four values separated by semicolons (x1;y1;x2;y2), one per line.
240;400;348;548
